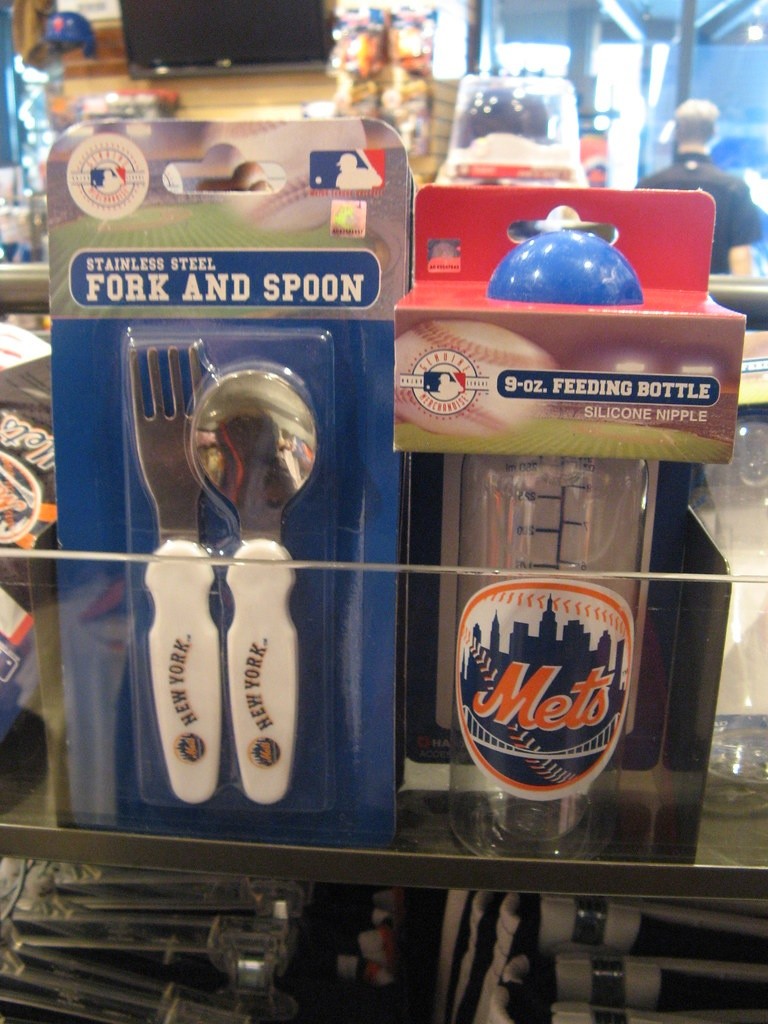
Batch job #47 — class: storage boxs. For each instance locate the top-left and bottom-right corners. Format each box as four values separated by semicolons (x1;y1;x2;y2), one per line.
47;116;748;864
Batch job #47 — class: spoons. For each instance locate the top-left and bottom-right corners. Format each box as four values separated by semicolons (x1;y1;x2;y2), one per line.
189;367;316;804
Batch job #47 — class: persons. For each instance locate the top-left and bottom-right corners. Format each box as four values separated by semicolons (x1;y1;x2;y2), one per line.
638;98;761;506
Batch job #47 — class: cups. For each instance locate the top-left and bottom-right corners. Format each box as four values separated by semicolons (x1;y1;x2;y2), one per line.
438;77;588;189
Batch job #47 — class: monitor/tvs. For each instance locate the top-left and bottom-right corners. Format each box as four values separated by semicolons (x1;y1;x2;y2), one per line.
120;0;328;78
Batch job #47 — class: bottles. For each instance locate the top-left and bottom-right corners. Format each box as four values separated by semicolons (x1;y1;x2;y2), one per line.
447;227;650;858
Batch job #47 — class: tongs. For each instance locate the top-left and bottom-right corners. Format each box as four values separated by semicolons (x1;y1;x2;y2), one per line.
0;864;309;1024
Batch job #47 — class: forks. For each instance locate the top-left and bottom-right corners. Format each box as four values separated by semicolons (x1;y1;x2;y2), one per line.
128;345;220;804
